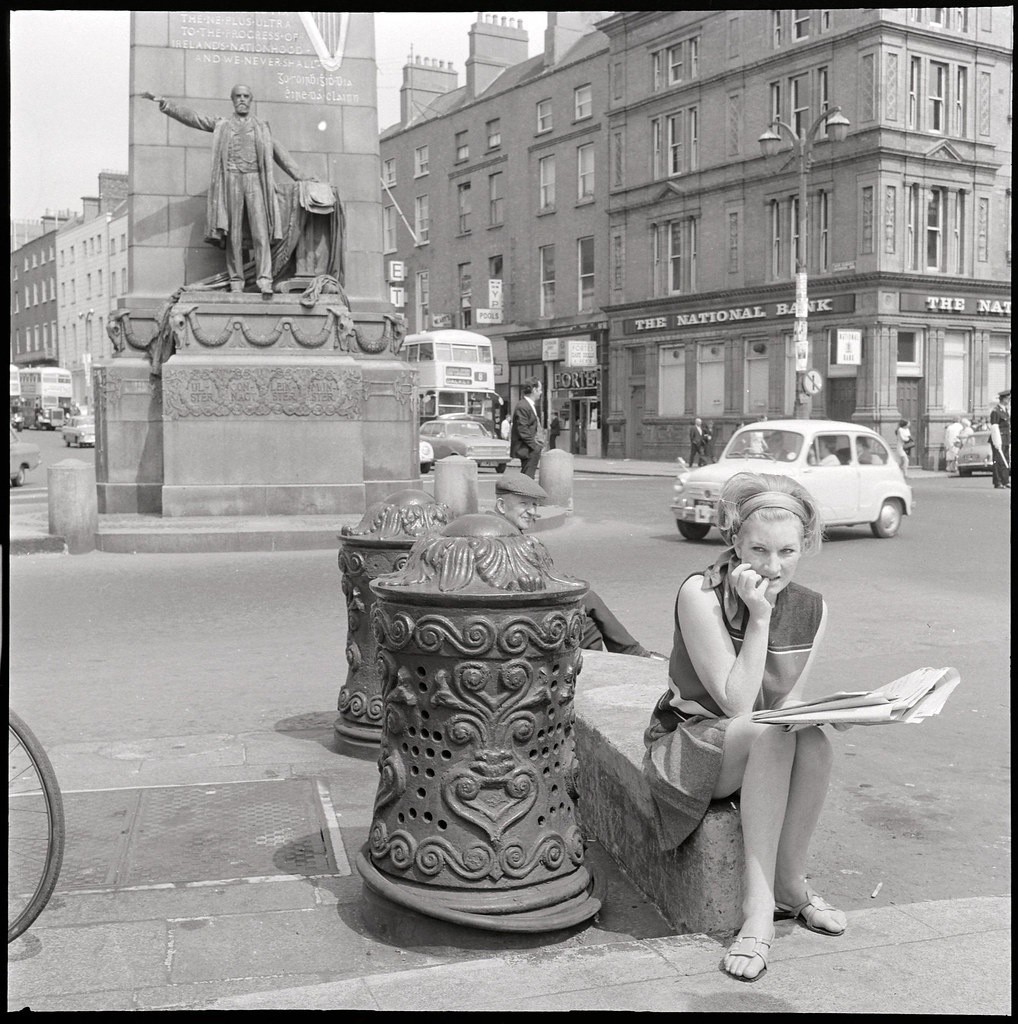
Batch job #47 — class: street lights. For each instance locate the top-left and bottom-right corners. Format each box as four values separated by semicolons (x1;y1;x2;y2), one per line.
757;101;849;414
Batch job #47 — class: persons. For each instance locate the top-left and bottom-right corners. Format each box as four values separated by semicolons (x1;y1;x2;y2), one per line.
142;82;319;298
511;375;546;479
701;419;718;466
487;471;668;661
10;394;89;431
689;418;708;467
500;414;511;441
550;411;560;450
638;468;848;983
729;389;1012;489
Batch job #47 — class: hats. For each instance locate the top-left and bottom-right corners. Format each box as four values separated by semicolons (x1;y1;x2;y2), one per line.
998;390;1011;396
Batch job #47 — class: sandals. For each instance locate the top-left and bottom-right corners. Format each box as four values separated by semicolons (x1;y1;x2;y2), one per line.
724;932;774;982
777;889;848;937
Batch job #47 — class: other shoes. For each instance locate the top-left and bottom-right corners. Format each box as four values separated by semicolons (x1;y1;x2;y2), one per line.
994;484;1011;488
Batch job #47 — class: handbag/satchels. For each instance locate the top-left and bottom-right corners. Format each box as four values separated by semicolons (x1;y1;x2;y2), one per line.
904;438;915;450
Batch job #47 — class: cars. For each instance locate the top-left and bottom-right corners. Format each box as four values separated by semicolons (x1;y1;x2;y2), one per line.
62;415;96;448
672;418;912;539
436;413;504;439
418;419;512;474
956;420;998;476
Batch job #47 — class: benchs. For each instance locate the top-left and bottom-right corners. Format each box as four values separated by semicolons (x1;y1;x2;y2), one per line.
566;648;746;937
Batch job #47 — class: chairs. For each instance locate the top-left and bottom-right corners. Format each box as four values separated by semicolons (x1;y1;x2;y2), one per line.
836;448;850;464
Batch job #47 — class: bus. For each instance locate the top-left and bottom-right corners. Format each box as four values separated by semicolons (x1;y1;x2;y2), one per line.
401;329;504;427
10;365;72;430
